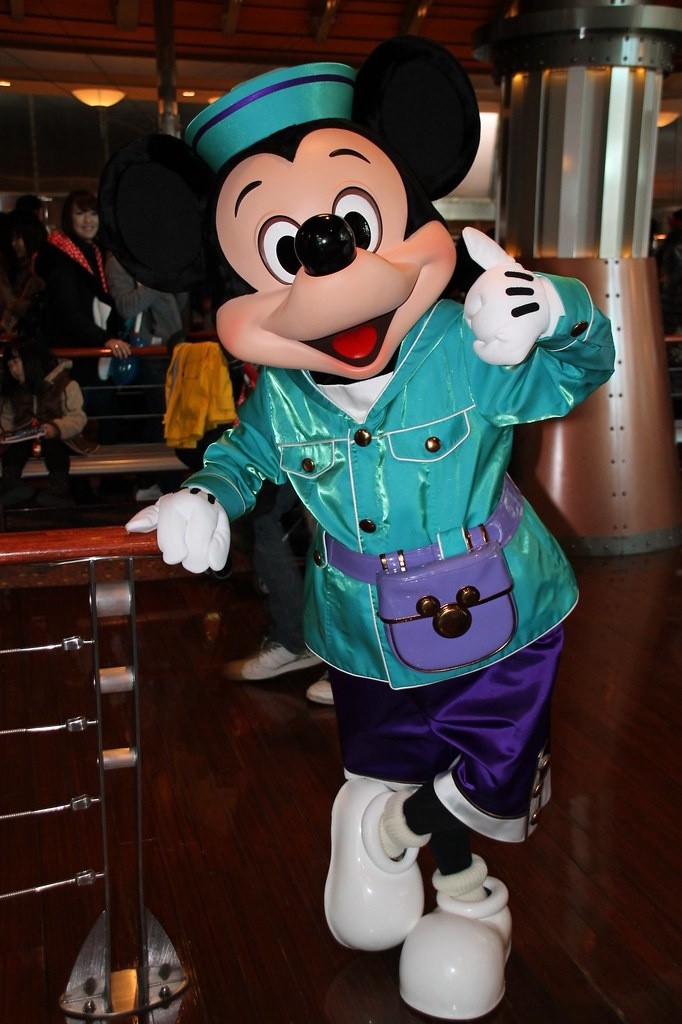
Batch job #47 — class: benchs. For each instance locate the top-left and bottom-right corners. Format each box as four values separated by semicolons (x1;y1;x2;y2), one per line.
0;525;197;1023
0;345;191;478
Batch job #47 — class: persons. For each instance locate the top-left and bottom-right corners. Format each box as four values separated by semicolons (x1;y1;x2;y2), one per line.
0;334;90;507
222;480;335;708
0;189;265;470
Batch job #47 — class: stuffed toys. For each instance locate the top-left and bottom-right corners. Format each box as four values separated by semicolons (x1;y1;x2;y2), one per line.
95;31;619;1021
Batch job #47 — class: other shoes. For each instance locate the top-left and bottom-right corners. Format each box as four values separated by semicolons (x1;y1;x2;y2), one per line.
136;484;164;501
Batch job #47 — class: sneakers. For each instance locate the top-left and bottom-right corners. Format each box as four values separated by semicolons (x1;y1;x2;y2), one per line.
223;643;324;680
306;670;334;704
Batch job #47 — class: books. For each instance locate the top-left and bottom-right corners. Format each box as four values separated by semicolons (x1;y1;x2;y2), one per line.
0;426;48;445
